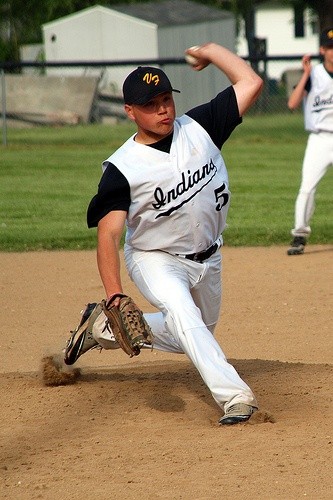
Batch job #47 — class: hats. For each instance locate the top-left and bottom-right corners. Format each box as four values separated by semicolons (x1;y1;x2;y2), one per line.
320;26;333;47
123;66;181;104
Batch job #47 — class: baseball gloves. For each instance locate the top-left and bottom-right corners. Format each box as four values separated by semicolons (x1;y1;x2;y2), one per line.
101;292;154;357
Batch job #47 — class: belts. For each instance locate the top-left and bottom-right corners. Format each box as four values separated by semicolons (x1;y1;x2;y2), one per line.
175;239;221;261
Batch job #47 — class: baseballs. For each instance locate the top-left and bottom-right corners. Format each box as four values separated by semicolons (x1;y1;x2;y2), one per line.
185;46;202;66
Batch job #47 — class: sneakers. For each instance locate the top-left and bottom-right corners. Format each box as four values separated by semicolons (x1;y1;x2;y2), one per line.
287;237;306;255
218;404;258;424
64;303;104;366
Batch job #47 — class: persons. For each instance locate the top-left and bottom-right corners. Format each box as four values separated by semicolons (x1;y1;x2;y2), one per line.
287;17;333;255
64;43;263;424
